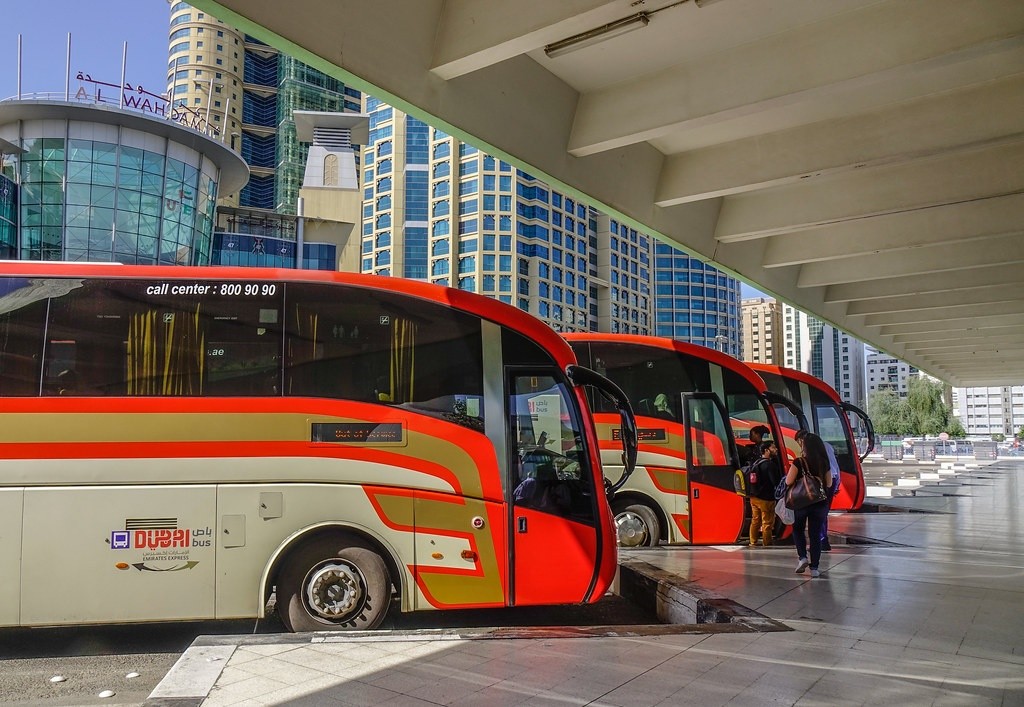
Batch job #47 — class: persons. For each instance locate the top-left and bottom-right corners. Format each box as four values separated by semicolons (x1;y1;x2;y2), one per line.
740;425;840;577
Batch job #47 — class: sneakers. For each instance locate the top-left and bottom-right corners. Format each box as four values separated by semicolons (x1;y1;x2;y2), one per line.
795;557;808;573
810;566;821;577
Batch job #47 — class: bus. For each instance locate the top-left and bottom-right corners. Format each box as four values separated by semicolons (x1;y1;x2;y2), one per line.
529;331;810;548
740;359;876;512
0;256;640;635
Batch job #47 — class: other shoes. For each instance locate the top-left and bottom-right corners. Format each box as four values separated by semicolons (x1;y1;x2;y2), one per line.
821;542;831;551
749;543;763;547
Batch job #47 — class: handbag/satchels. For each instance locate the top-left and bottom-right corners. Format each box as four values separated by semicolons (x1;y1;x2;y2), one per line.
775;497;795;524
783;456;828;510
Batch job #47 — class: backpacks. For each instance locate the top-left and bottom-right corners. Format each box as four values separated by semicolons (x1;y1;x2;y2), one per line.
734;459;768;496
